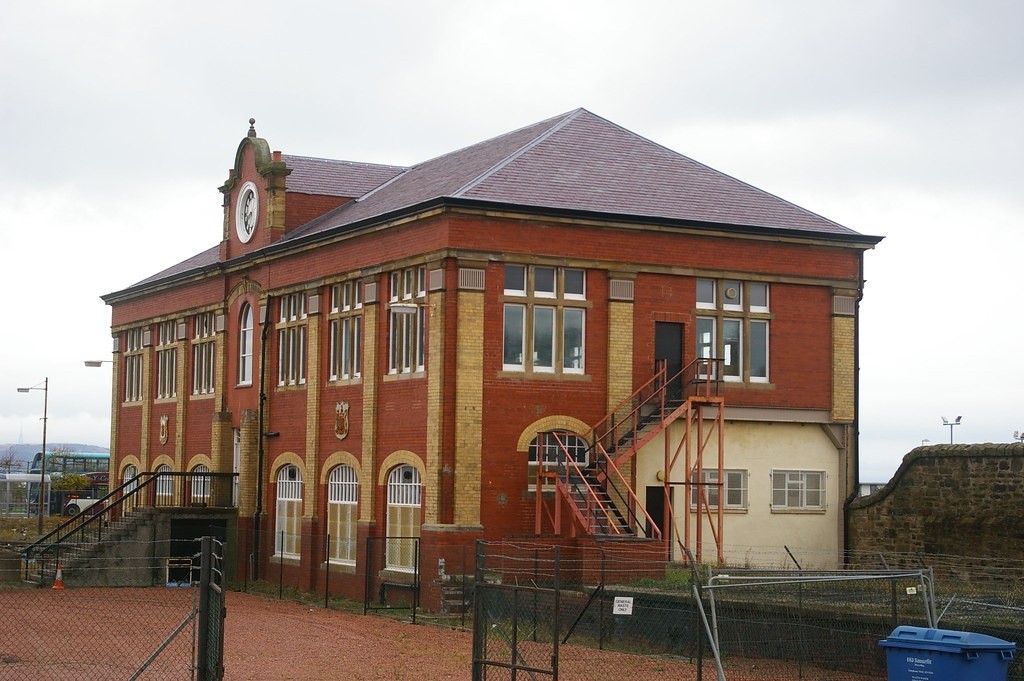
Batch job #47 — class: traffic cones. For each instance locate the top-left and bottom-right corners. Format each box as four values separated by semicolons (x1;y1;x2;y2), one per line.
52;562;65;590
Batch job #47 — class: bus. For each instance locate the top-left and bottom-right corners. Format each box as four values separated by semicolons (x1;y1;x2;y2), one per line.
25;452;110;518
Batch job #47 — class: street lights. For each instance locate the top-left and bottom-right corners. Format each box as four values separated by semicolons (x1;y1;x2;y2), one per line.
17;377;48;536
941;416;962;444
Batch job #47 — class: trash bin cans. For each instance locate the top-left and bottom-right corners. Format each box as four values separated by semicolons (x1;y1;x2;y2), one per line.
877;625;1018;681
